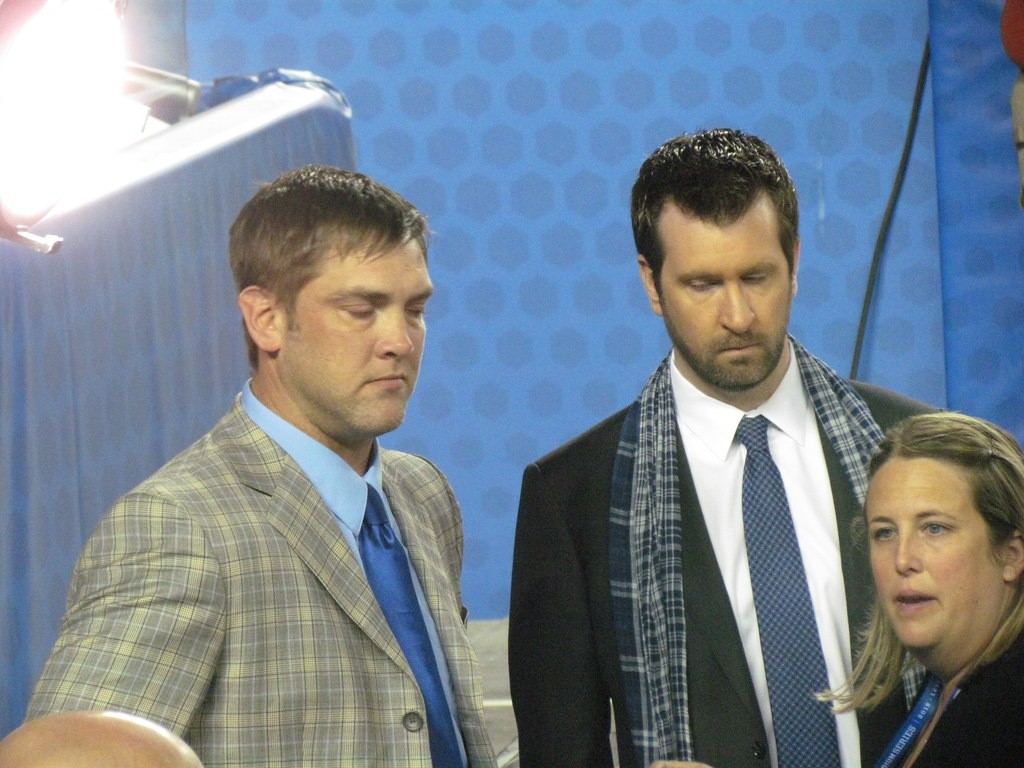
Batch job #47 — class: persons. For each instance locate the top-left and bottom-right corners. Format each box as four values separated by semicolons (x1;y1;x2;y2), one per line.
0;169;499;768
643;412;1024;768
508;127;945;768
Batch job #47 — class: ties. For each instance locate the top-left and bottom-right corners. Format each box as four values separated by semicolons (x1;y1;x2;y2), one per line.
733;415;841;768
356;483;465;768
